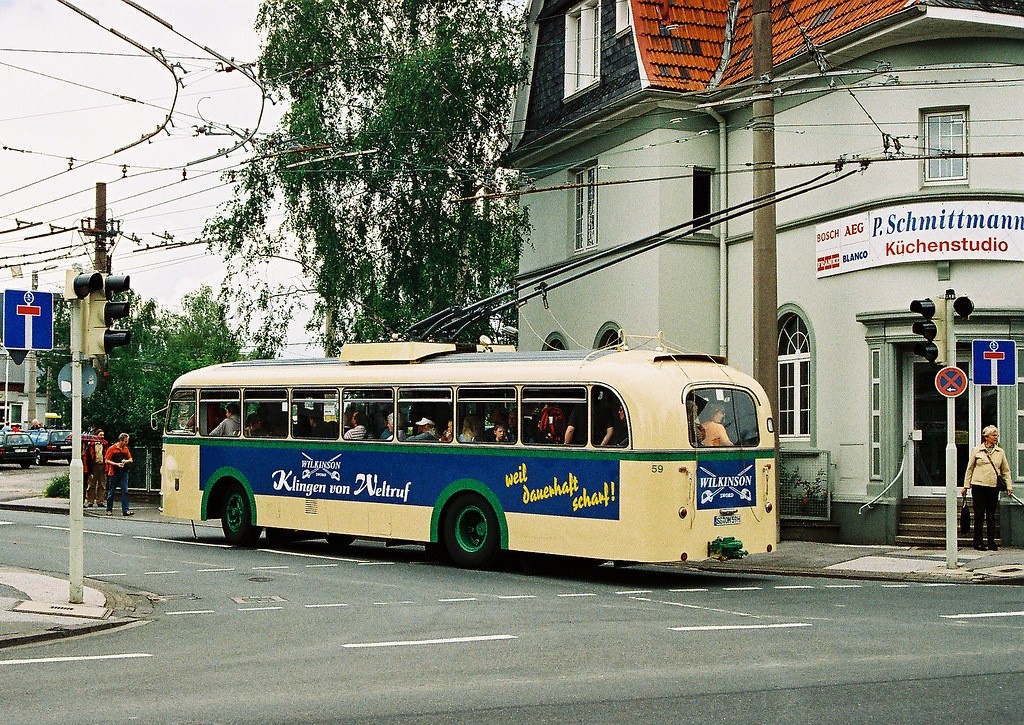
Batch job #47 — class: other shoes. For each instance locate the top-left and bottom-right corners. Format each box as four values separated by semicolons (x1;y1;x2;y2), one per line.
107;511;112;516
88;504;93;507
98;504;105;507
988;544;998;551
974;544;987;551
123;511;134;516
83;505;87;508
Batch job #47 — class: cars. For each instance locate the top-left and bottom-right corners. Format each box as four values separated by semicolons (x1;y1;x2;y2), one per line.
0;425;72;469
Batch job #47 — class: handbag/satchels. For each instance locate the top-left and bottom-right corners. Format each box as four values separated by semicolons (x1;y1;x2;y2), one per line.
997;476;1007;492
960;496;971;534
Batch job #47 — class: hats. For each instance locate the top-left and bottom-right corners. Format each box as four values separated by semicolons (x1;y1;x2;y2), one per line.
415;417;436;426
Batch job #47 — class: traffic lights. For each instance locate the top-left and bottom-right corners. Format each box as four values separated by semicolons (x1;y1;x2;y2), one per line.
910;298;947;363
953;296;974;316
65;269;104;301
85;272;130;358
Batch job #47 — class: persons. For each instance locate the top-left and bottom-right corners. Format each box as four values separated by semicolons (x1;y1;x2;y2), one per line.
961;424;1013;551
29;419;40;430
81;429;109;507
208;403;338;438
529;389;629;446
103;433;134;516
346;404;520;444
699;402;735;447
180;412;195;431
39;421;44;427
12;424;20;432
2;422;11;432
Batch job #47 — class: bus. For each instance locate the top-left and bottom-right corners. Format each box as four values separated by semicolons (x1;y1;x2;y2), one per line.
147;159;876;571
